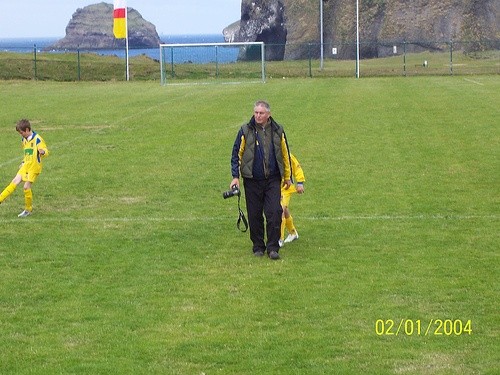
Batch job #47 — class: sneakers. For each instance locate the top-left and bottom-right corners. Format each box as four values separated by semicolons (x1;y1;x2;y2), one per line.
18;210;33;218
255;251;264;256
268;250;279;259
279;241;284;248
284;234;298;243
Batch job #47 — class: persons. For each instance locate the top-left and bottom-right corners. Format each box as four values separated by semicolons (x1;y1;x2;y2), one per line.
278;152;306;248
230;101;292;260
0;119;48;218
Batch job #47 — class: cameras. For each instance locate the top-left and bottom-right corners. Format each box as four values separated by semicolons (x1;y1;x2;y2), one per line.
223;184;241;199
